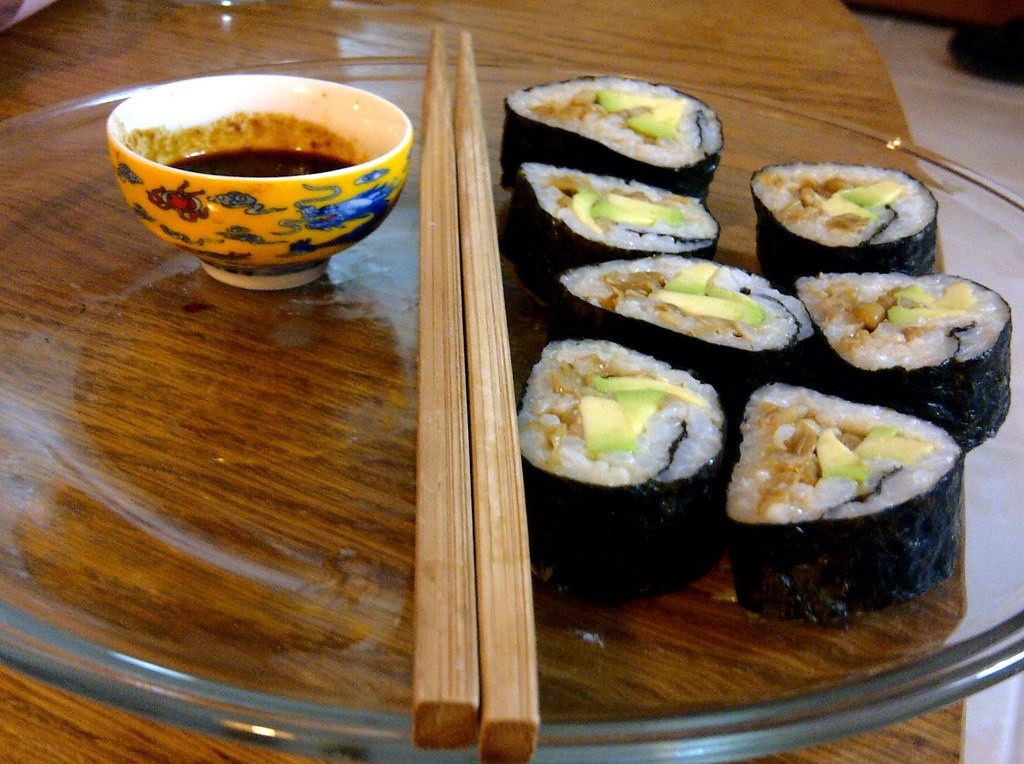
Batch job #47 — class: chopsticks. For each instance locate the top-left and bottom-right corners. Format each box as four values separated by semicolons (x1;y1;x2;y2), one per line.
416;22;539;762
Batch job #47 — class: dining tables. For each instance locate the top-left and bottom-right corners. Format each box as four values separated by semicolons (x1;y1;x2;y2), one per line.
0;0;966;764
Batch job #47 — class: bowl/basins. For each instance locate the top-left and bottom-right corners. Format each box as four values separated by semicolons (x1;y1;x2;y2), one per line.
103;73;413;290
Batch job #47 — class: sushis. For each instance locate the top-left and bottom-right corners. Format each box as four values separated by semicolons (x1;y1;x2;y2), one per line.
498;76;1014;623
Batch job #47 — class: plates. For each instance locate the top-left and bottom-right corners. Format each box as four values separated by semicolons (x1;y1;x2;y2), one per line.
0;64;1023;764
